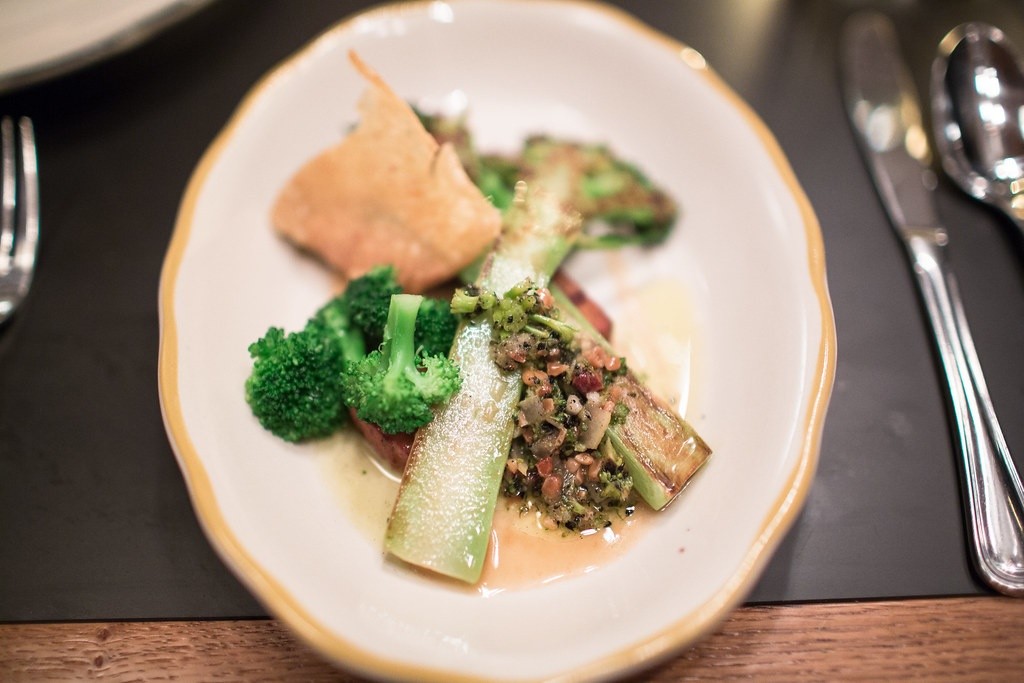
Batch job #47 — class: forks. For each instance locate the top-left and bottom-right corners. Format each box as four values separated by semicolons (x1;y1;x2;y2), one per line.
0;117;39;322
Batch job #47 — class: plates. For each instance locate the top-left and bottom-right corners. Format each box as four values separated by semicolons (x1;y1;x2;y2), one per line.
0;0;210;92
157;0;838;683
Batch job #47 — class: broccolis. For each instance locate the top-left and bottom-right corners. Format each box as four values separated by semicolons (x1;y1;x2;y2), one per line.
337;267;457;353
247;311;364;444
340;294;463;438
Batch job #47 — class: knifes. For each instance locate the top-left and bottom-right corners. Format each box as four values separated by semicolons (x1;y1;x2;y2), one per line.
839;12;1024;597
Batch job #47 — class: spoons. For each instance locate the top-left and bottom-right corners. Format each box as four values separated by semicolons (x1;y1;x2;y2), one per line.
932;21;1024;232
